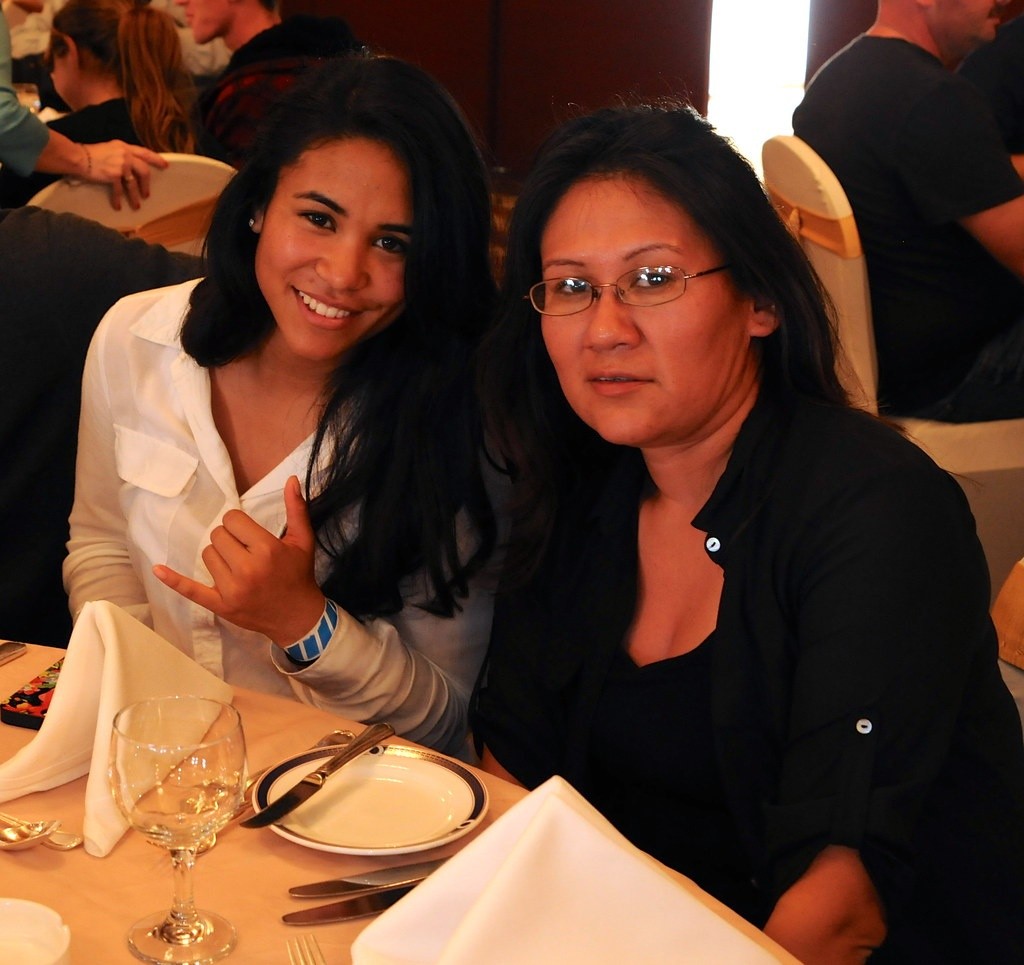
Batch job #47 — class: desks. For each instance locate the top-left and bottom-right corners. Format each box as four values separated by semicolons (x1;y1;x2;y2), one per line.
0;637;805;965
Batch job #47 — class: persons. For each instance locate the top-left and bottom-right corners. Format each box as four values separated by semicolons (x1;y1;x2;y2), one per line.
62;53;513;770
0;0;367;211
476;101;1024;965
791;0;1024;423
0;203;228;649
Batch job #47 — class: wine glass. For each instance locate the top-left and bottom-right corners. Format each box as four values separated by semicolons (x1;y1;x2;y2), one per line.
109;696;247;965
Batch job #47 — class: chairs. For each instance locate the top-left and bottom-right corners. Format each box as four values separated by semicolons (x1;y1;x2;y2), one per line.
26;152;236;259
762;135;1024;610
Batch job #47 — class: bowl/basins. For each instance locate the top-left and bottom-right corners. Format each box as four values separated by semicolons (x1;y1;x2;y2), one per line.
0;898;70;965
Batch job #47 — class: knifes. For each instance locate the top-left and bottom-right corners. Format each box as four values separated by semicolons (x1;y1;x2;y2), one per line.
282;885;417;925
241;722;394;829
288;857;453;897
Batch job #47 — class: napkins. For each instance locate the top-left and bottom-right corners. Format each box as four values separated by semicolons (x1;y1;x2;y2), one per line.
350;775;803;965
0;600;233;859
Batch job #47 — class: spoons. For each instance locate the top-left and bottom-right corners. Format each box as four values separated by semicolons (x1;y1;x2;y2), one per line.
180;730;358;816
0;821;61;850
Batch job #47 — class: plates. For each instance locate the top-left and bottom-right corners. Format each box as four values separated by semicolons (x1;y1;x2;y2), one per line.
251;743;489;856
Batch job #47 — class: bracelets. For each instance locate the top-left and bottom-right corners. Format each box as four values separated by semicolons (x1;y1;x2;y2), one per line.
64;142;92;189
283;595;339;668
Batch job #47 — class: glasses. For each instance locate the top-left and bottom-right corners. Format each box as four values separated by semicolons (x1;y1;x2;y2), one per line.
522;264;731;316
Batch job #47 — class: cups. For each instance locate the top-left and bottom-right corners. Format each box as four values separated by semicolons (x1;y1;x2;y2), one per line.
11;84;40;116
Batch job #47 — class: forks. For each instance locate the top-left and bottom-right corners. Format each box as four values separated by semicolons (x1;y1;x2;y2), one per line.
131;801;253;856
285;933;328;965
